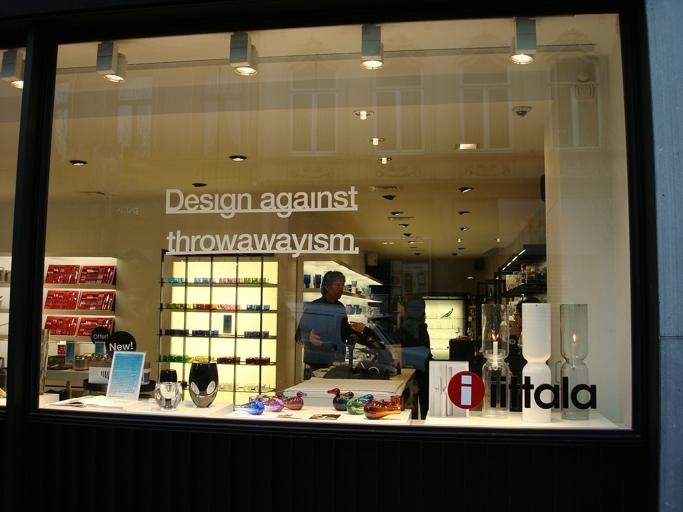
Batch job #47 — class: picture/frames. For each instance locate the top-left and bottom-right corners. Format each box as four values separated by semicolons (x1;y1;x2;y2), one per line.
101;350;145;401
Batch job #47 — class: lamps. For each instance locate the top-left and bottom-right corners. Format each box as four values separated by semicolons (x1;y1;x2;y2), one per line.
0;17;595;91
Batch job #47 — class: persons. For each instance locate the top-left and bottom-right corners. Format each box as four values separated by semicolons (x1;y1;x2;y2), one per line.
509;295;541;377
293;269;387;381
349;293;430;420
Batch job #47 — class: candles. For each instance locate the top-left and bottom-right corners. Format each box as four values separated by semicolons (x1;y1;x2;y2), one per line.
569;334;580;360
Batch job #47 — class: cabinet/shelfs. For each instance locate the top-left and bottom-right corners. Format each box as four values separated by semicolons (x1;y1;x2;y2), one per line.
294;256;383;381
496;244;546;359
0;249;116;399
154;248;291;403
